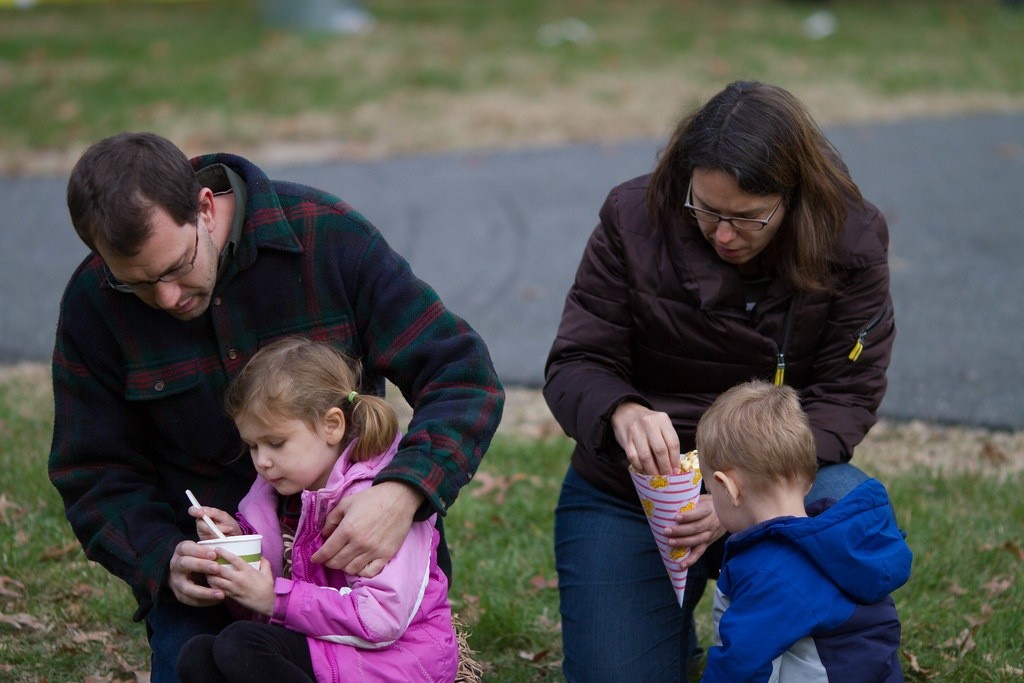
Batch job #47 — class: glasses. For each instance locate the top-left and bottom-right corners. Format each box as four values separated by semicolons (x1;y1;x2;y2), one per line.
103;212;199;292
684;176;785;232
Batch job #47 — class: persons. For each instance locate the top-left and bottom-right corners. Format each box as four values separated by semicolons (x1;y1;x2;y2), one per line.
541;80;896;683
187;336;458;683
47;132;506;683
697;381;912;683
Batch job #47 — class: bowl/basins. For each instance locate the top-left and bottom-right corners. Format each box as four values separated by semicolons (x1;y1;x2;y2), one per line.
196;534;263;577
